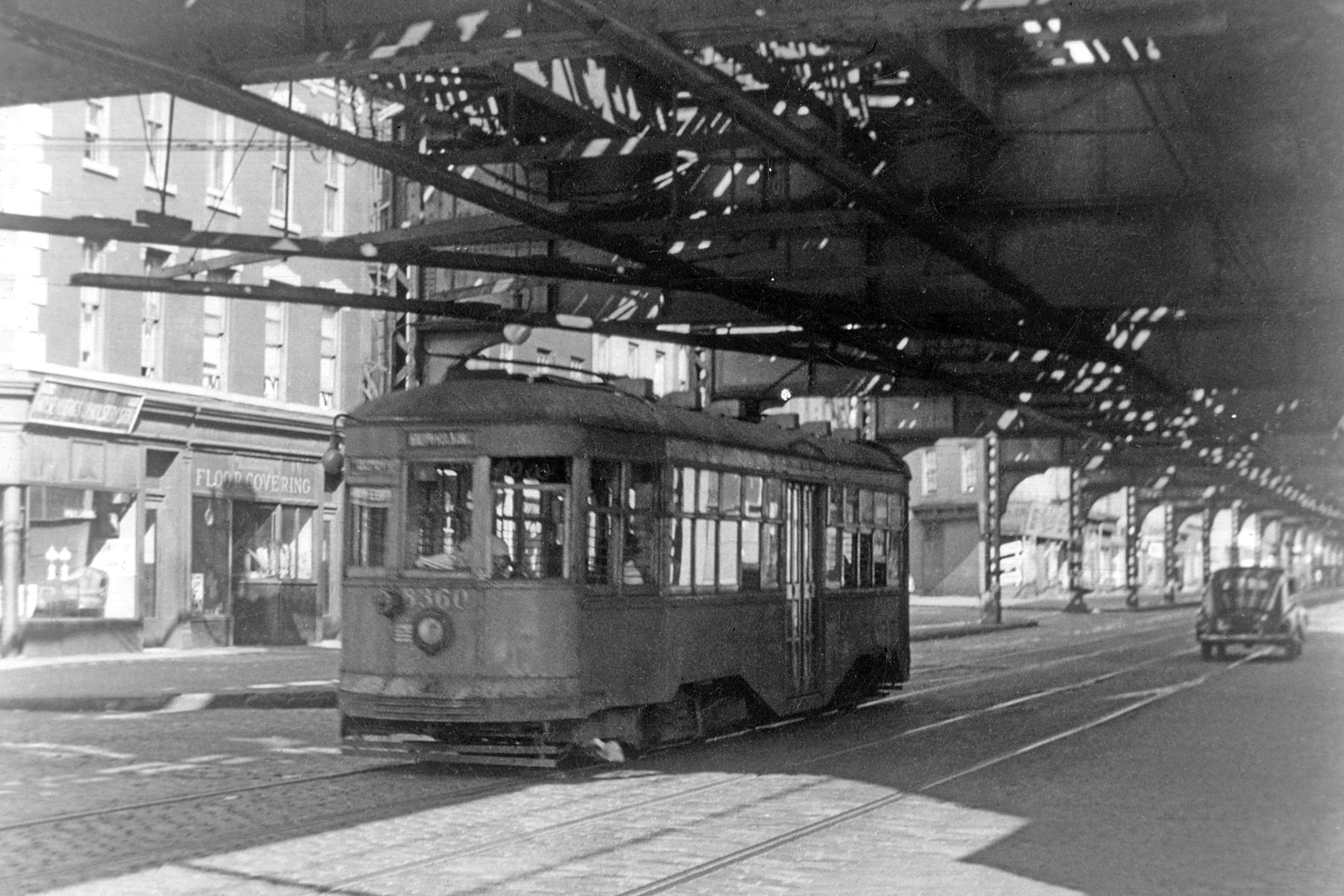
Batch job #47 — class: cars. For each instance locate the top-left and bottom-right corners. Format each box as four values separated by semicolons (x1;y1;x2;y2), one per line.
1193;566;1308;662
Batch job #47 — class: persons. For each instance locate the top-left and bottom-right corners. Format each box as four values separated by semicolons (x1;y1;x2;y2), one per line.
453;533;509;580
623;533;644;585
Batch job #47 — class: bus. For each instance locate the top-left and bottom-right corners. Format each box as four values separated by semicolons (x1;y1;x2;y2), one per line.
321;347;912;767
